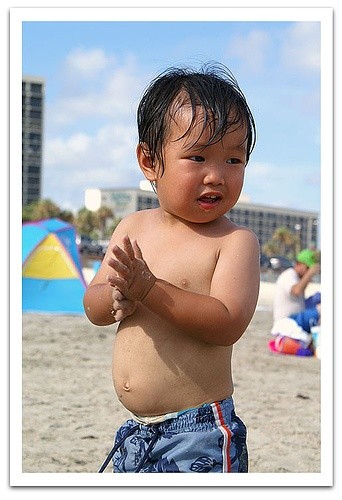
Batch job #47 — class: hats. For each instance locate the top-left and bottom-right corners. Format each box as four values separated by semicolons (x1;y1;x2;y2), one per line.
297;249;317;268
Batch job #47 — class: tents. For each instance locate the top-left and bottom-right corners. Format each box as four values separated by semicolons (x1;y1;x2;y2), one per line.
22;218;88;316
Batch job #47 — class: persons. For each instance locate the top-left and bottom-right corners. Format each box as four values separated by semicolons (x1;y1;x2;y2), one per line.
270;250;320;329
84;60;261;472
